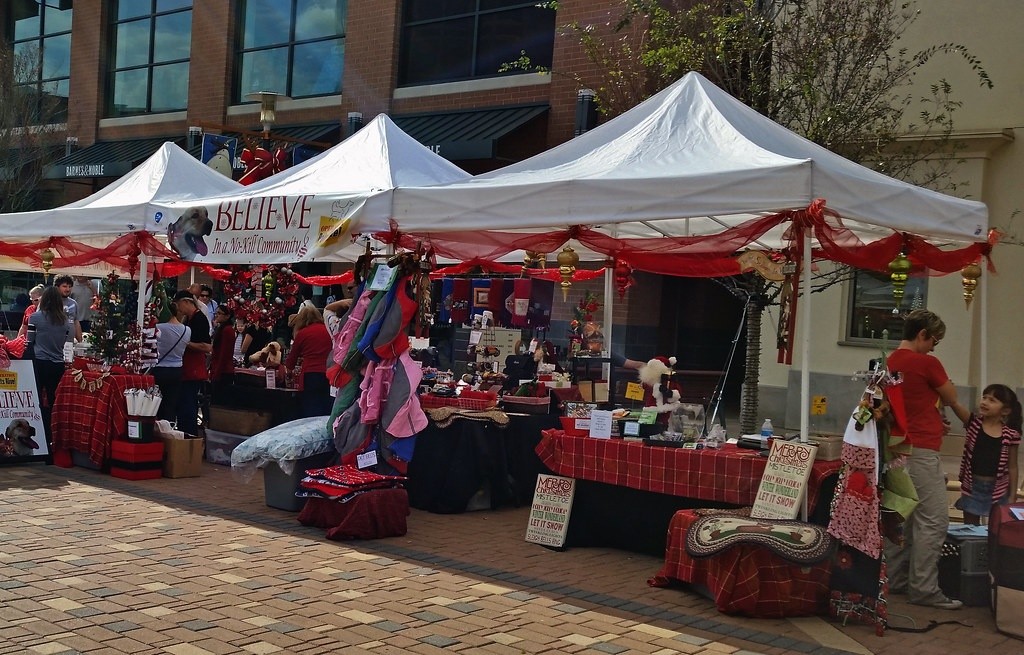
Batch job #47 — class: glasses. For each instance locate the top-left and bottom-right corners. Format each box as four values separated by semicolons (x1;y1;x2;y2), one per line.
214;312;226;315
926;332;940;346
200;294;209;297
30;296;42;302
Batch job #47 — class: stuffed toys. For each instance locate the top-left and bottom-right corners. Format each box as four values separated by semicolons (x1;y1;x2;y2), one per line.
639;356;682;427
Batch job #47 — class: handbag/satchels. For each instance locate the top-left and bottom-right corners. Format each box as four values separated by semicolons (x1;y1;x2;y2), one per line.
325;273;435;477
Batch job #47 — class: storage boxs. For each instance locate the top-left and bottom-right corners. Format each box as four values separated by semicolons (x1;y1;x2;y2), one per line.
938;537;989;608
205;404;273;466
559;417;591;437
618;420;666;438
265;451;338;511
502;395;551;414
807;431;843;461
579;379;609;403
112;433;203;481
519;380;571;388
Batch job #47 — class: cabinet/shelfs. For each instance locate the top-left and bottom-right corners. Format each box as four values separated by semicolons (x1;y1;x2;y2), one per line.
476;330;521;374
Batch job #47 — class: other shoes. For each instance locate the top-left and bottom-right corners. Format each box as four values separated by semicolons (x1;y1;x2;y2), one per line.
197;420;209;431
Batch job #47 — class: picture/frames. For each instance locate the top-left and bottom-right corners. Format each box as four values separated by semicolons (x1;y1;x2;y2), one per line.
474;288;490;307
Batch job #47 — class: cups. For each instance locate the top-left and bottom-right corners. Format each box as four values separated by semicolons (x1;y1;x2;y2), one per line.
474;311;493;331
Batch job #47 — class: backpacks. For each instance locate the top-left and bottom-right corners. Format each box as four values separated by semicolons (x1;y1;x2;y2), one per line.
503;355;535;389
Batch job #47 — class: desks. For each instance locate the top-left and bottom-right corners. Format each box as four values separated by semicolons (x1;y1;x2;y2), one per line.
213;385;305;428
50;369;155;472
535;428;843;557
403;411;564;514
647;509;833;618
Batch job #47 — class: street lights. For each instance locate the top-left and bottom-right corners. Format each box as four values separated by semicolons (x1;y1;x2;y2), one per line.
245;91;292;153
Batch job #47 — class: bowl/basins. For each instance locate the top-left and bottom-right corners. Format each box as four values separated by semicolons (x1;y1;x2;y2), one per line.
559;417;590;437
87;364;104;372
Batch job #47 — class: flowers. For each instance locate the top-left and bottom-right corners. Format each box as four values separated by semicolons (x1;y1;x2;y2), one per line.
565;288;604;335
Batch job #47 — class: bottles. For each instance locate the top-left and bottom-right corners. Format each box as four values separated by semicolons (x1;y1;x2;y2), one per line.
761;419;774;449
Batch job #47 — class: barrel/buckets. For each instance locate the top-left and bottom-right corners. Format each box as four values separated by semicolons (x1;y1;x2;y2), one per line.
126;416;157;443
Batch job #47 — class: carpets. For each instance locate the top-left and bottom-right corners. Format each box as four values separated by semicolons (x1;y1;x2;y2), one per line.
685;515;833;564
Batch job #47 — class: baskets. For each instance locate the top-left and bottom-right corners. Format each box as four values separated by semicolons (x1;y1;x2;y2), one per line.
419;397;496;409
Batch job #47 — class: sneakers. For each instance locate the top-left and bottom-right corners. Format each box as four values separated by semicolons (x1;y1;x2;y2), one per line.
900;587;908;594
931;598;962;609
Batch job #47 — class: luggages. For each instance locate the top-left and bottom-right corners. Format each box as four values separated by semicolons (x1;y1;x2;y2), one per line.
988;501;1024;640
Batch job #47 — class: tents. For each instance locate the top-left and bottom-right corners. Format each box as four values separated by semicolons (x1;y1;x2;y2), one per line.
1;142;248;325
145;112;478;324
391;71;993;411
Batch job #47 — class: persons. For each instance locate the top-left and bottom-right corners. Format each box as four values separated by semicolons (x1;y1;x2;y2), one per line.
952;385;1024;526
611;351;644;401
880;308;978;608
18;273;358;440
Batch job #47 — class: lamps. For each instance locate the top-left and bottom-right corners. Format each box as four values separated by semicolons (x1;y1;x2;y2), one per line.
187;126;202;150
66;136;78;156
573;88;597;136
348;111;362;139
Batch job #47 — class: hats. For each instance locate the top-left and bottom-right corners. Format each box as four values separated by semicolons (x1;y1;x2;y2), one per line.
173;289;195;301
